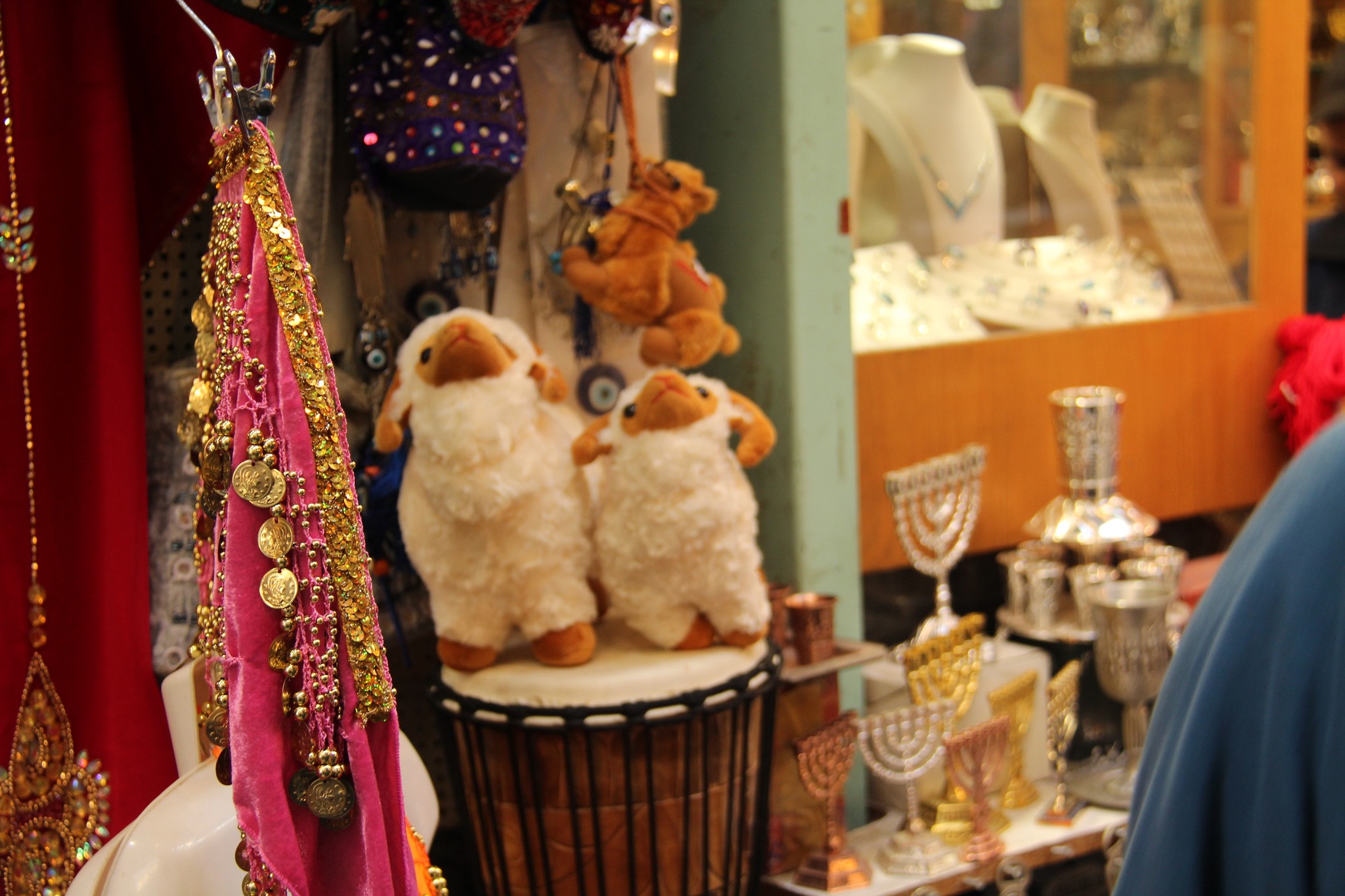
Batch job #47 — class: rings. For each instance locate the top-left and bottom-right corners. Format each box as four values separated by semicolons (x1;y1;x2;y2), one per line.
847;223;1169;342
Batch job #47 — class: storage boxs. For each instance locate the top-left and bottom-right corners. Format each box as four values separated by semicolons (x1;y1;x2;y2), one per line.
765;636;886;873
864;640;1052;813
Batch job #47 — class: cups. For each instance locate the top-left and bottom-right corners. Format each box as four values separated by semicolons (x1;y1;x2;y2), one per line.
767;582;792;648
995;538;1188;627
783;592;839;666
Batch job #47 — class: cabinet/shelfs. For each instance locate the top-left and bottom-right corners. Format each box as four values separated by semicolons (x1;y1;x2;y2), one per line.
1018;0;1345;213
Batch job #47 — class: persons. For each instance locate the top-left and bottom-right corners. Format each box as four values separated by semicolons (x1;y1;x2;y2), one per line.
1110;412;1345;896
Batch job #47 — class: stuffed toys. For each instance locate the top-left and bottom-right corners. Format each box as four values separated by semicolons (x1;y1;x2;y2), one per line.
373;307;599;673
571;363;778;650
560;161;740;371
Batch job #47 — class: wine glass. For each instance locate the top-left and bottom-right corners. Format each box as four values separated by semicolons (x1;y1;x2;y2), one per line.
1089;584;1171;786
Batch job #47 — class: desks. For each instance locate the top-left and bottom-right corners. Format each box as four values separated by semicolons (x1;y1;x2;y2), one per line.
755;756;1130;895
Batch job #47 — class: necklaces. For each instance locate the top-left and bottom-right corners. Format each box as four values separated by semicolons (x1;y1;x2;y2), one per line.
1055;121;1121;199
895;103;994;271
